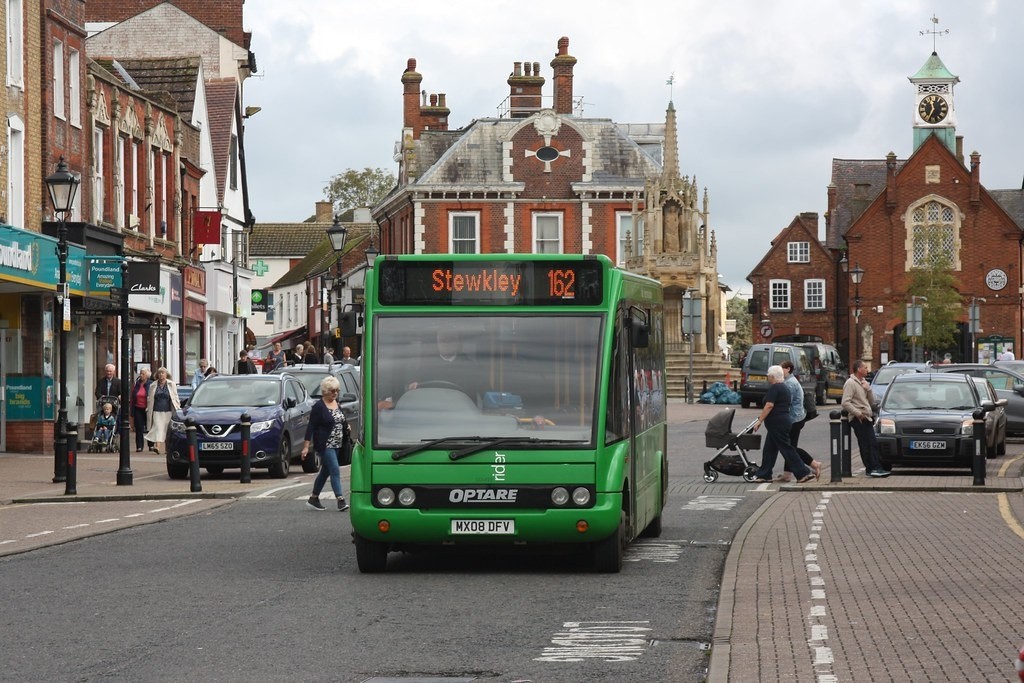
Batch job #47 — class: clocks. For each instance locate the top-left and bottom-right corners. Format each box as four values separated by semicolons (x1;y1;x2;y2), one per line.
918;94;949;124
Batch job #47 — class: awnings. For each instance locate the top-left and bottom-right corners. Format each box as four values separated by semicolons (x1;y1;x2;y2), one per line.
258;325;306;348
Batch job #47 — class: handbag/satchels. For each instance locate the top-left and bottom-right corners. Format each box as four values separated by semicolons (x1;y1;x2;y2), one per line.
803;392;820;423
336;420;353;466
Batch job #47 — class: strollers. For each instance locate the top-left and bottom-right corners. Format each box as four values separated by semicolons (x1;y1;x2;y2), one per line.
703;408;762;483
86;395;121;454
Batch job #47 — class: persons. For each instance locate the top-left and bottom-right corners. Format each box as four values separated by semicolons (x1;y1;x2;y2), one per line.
774;361;821;483
942;359;950;364
301;376;352;512
234;341;361;375
93;358;221;454
377;324;478;410
842;360;891;477
752;365;814;484
1001;348;1015;361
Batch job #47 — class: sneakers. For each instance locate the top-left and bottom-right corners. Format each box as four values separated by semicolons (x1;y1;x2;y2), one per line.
306;496;326;511
337;500;350;511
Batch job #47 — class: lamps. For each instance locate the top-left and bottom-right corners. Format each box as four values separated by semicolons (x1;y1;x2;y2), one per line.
241;106;261;118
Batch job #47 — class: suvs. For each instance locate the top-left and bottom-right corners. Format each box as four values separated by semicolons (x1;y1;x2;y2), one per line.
165;373;320;479
268;359;361;466
870;360;935;402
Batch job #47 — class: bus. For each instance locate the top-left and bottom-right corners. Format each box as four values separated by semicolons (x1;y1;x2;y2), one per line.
339;252;670;574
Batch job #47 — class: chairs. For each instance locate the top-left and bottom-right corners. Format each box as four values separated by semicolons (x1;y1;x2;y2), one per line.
895;388;922;408
940;388;964;407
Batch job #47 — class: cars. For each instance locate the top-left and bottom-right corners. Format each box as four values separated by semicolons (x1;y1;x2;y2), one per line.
992;359;1024;378
873;372;988;475
933;363;1024;435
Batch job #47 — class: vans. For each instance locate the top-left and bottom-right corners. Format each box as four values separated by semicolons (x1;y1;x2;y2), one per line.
741;343;818;407
781;341;847;405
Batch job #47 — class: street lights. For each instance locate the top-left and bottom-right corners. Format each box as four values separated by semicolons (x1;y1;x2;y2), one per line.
972;297;986;363
850;261;864;361
325;214;347;359
46;155;80;484
912;295;927;363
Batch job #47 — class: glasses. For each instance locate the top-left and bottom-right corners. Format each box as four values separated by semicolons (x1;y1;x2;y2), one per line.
329;387;341;394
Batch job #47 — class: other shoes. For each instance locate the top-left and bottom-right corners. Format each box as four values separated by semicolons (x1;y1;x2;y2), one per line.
866;468;891;476
93;438;98;443
813;462;821;482
149;447;154;451
773;475;791;483
754;476;773;482
136;448;143;452
102;440;108;444
153;447;159;454
797;473;815;484
